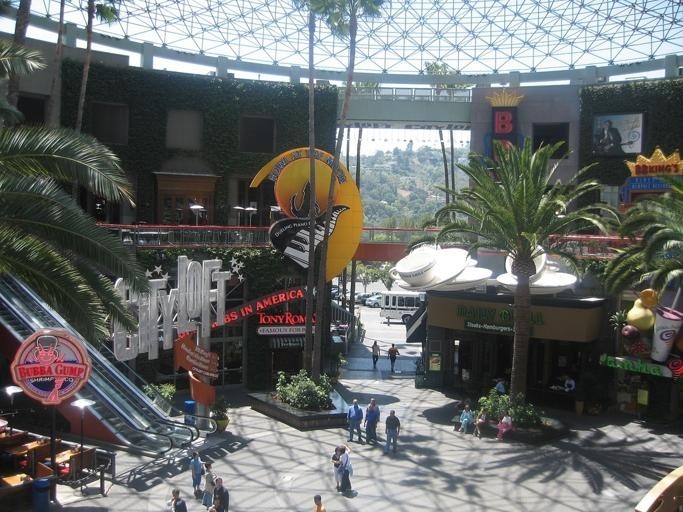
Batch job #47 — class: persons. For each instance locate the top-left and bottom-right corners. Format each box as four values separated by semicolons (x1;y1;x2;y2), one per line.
388;344;400;371
167;452;229;512
311;495;326;512
599;120;625;152
459;373;585;442
331;398;401;498
372;340;380;369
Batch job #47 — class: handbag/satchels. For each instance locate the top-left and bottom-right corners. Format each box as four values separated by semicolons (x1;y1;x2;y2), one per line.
200;467;205;475
336;463;343;474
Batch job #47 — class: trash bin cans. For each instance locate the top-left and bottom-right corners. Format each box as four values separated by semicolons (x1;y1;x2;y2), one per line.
184;399;196;426
31;479;50;512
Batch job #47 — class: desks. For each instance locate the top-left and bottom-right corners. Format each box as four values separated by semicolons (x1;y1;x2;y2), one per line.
0;430;89;512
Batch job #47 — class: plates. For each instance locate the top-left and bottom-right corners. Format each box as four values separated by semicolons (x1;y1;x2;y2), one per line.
437;266;493;291
393;248;472;290
497;271;577;295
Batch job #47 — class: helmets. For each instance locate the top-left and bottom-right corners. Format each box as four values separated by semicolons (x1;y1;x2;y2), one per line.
193;451;200;456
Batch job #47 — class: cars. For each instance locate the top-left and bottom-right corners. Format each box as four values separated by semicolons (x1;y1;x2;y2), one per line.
330;285;383;310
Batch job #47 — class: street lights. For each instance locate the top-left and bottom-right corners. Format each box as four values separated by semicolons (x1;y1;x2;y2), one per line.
5;383;23;437
69;398;95;476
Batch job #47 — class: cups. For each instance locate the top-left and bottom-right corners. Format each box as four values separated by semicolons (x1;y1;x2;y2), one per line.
386;253;436;286
650;309;682;364
505;243;548;282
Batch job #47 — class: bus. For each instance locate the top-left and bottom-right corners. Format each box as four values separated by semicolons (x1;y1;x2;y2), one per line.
378;290;426;326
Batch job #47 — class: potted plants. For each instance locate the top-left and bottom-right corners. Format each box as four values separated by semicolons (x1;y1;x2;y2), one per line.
209;395;231;433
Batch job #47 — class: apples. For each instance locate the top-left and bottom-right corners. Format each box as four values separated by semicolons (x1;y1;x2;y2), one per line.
641;288;657;308
622;325;640;338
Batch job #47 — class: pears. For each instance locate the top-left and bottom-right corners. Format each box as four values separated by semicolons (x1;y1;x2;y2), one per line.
627;298;654;330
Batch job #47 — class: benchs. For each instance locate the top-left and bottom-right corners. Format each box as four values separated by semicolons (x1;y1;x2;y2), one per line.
61;448;96;480
